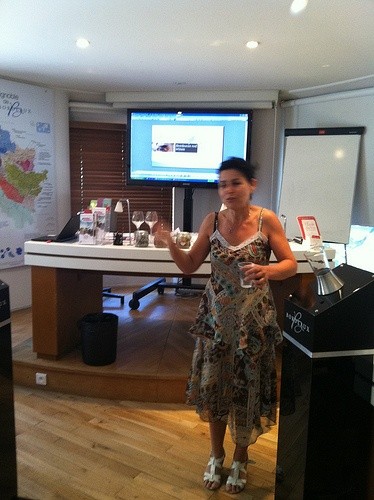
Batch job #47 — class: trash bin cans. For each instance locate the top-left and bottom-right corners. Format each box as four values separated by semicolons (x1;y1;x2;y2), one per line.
81;313;119;366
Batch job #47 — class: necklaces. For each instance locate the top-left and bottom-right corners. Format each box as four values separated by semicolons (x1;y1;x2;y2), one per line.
229;206;253;234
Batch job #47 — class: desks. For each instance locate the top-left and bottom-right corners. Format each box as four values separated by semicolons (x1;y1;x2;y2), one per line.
23;230;337;357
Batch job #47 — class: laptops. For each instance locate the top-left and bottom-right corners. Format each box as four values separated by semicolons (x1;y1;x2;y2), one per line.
31;215;80;242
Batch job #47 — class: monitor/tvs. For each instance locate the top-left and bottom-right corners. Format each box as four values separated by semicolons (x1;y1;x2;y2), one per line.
125;107;253;189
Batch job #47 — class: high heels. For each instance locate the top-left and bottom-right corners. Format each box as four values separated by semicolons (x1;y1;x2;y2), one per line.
226;455;256;493
203;450;226;490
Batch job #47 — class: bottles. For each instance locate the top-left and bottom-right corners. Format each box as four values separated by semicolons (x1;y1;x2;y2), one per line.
176;232;190;249
133;230;149;247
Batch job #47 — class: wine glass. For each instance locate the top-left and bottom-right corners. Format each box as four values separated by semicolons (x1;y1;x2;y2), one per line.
131;211;145;230
145;211;158;239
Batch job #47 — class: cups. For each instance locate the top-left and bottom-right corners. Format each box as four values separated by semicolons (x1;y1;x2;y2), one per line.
238;262;253;288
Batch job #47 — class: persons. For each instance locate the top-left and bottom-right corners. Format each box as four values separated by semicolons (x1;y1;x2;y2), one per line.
154;157;298;493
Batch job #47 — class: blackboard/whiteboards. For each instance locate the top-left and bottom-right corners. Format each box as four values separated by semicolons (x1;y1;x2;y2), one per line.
277;134;362;244
